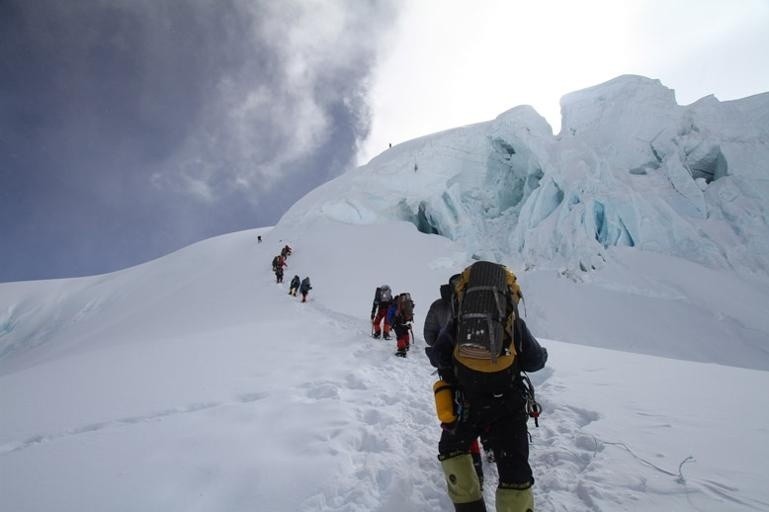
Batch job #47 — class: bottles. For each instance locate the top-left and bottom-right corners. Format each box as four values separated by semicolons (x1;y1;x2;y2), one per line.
433;379;458;424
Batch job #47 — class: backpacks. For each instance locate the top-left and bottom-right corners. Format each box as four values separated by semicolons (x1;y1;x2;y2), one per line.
395;292;414;326
379;284;393;303
451;259;524;377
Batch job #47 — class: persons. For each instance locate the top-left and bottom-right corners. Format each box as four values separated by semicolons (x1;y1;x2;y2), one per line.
423;261;548;512
370;285;394;341
271;243;294;283
300;277;312;303
288;274;300;297
387;292;415;358
257;235;262;244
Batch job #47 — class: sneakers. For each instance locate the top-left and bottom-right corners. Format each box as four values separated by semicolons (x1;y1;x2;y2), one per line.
373;331;381;337
395;350;406;356
382;331;390;338
